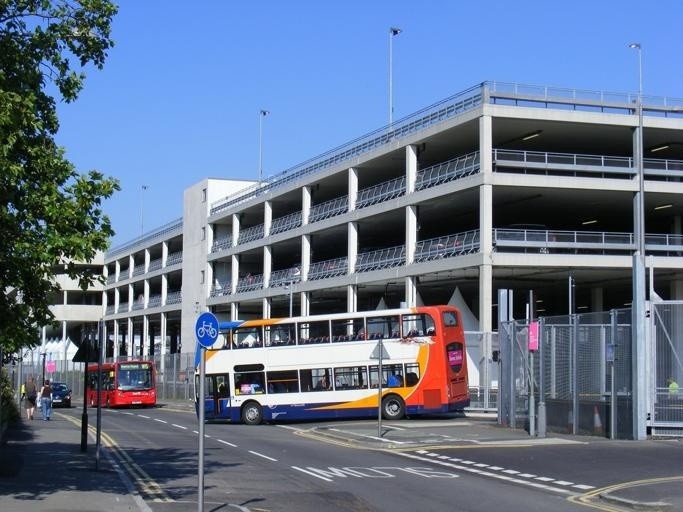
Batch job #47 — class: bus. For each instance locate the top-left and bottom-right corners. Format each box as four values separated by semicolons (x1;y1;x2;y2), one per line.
190;303;472;426
84;359;157;410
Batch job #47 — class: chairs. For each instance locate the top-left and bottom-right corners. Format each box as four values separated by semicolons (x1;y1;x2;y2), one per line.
283;381;379;394
217;328;436;351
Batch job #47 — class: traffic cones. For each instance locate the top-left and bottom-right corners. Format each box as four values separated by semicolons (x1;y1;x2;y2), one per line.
590;405;604;436
566;404;573;433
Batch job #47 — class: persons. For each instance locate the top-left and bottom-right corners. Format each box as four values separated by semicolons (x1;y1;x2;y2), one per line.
246;271;254;285
289;264;301;284
667;376;679;400
38;380;53;421
236;376;242;389
24;376;37;420
20;381;27;400
217;378;225;392
321;374;326;387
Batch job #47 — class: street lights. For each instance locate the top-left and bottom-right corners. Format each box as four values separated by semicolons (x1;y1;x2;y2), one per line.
10;359;16;388
38;352;46;389
628;41;644;107
257;107;271;183
138;184;151;236
387;25;402;130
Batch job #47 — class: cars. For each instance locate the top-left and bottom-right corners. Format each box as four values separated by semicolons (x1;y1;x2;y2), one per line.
43;382;72;408
